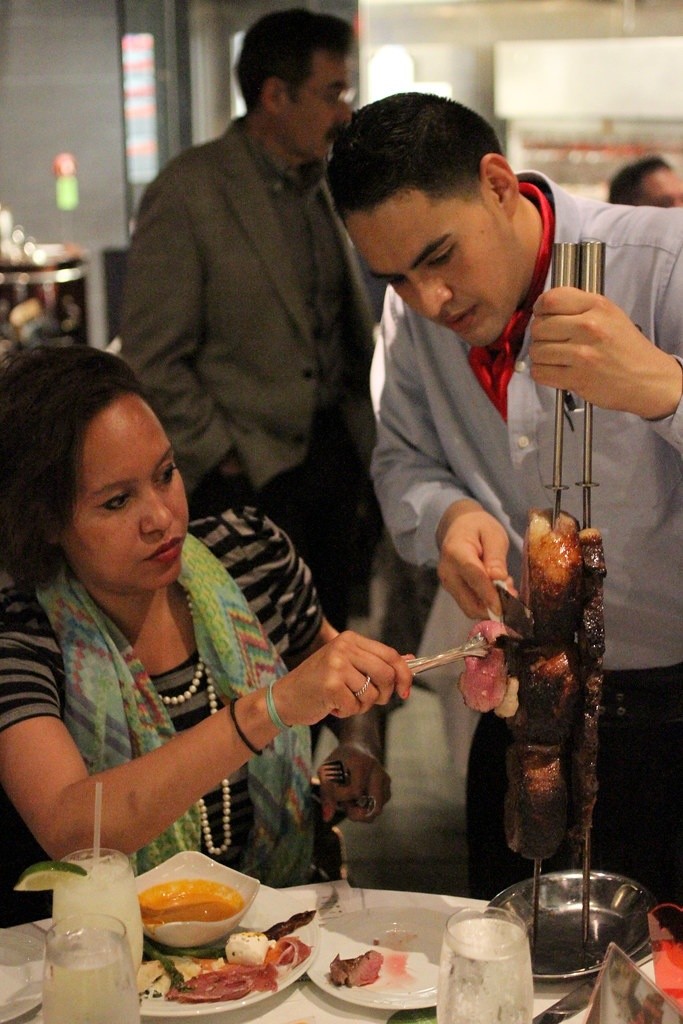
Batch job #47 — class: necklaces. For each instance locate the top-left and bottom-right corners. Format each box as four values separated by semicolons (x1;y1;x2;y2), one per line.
158;588;230;855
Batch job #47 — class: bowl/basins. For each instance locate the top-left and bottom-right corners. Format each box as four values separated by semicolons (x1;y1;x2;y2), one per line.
485;868;656;979
134;851;260;948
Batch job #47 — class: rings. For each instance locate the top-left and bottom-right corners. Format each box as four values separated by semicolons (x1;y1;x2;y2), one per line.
354;675;372;695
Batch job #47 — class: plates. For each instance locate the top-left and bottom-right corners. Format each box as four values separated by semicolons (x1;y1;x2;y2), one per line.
0;929;45;1023
140;883;321;1016
304;904;463;1009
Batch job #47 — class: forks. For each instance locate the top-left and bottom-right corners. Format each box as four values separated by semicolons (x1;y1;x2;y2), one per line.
322;760;350;785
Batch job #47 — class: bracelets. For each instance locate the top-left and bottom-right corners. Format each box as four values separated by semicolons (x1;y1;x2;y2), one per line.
230;698;265;757
268;680;293;731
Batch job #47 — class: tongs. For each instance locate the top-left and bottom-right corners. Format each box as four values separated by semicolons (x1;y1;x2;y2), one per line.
404;630;487;675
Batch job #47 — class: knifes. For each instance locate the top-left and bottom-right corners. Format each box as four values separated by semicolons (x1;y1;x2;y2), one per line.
491;582;534;638
532;921;683;1024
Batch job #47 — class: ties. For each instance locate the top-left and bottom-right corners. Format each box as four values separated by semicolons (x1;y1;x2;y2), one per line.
465;181;555;417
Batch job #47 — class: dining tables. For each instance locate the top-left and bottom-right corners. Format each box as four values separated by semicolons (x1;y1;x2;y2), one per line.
0;888;655;1024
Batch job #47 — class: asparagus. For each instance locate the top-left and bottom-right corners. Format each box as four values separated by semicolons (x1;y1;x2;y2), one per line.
142;909;317;989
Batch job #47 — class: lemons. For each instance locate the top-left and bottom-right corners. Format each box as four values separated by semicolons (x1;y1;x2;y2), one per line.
13;861;88;891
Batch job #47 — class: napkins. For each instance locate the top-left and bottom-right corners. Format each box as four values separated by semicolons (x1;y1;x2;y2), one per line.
277;879;354;927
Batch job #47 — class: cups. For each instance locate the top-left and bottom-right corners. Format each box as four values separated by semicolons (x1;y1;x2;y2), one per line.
437;906;532;1024
53;848;144;977
41;914;139;1024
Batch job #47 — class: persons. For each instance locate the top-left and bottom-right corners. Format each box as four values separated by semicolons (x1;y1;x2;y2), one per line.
112;10;392;764
0;341;416;935
610;157;683;209
325;93;683;944
